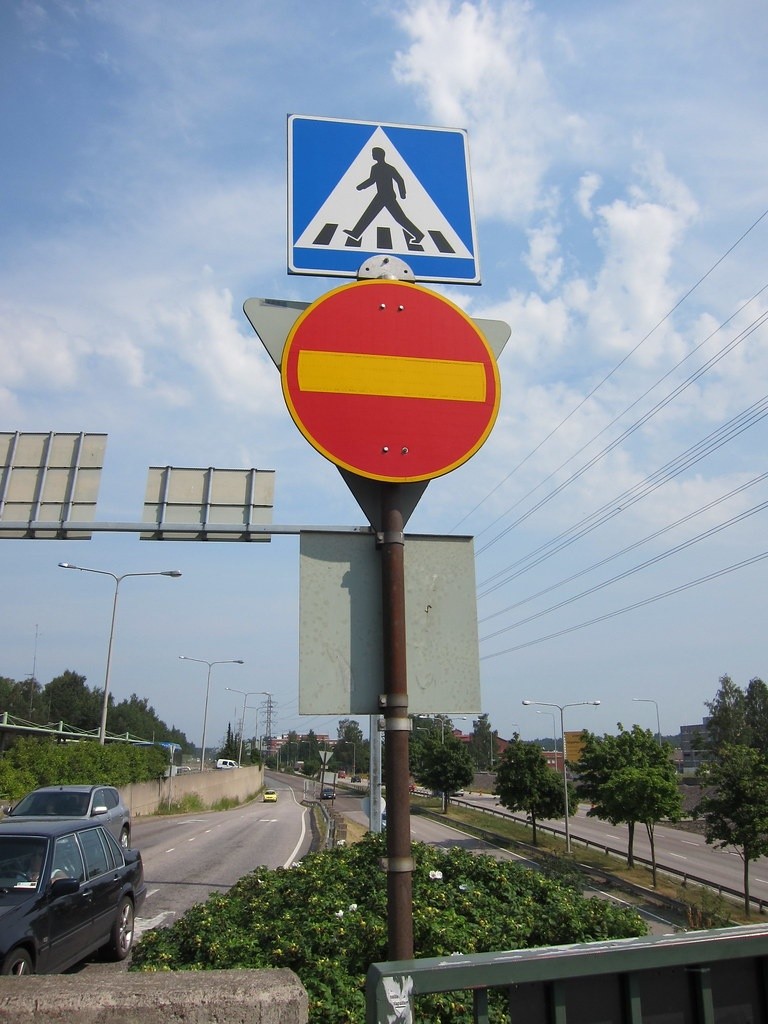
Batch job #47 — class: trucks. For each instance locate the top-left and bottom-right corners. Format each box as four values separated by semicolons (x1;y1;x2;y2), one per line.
293;761;305;771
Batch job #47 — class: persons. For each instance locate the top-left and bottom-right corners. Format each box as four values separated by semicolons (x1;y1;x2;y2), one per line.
25;845;69;883
78;795;94;815
45;800;56;814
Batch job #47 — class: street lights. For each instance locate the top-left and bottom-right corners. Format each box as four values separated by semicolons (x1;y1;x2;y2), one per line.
632;696;662;746
418;715;469;814
225;687;270;769
58;561;182;746
520;699;602;856
178;655;245;773
536;711;559;773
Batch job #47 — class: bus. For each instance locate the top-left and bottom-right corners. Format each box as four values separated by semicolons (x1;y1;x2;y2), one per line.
136;742;183;774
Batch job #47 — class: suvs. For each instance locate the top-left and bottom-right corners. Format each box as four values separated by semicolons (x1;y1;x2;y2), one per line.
1;784;133;849
451;785;465;796
216;759;242;769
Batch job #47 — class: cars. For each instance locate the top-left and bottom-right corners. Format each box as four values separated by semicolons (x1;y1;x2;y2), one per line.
263;789;278;802
351;776;361;783
0;819;147;977
338;771;346;779
320;788;336;799
409;783;416;793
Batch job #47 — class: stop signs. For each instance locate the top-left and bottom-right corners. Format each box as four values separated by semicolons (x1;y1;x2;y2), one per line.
280;279;502;483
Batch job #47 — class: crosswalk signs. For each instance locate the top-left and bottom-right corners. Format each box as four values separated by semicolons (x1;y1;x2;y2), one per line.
285;110;483;287
